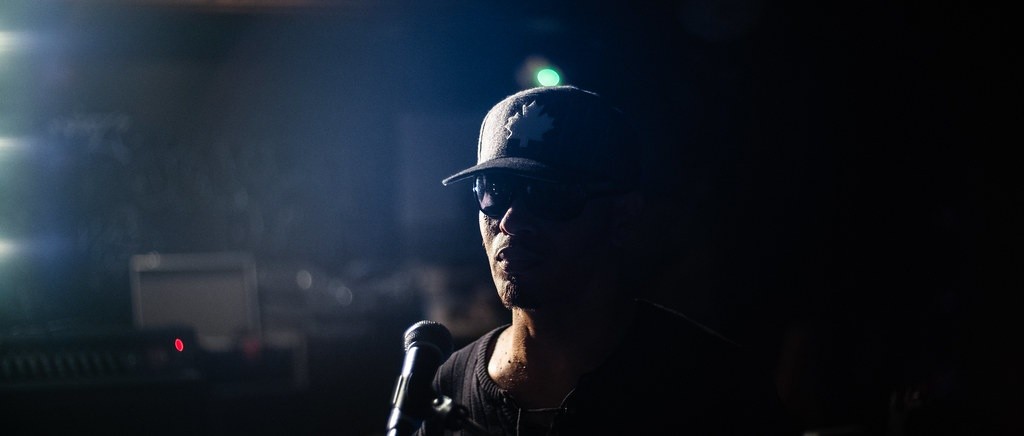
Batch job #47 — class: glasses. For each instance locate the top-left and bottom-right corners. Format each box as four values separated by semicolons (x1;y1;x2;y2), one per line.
473;174;632;220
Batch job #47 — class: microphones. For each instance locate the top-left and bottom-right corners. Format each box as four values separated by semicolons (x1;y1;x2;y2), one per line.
385;320;455;436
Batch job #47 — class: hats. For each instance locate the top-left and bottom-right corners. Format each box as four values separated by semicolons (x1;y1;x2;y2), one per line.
442;86;648;192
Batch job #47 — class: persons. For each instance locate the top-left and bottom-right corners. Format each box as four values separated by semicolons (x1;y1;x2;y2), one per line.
775;285;1024;436
420;87;807;436
